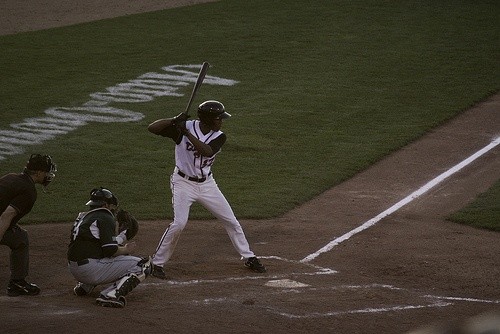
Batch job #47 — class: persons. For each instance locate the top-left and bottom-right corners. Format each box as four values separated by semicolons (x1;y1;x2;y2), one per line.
68;188;151;307
148;102;266;279
0;153;56;297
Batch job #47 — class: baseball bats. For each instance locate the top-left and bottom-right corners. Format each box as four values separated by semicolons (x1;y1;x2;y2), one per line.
185;61;211;114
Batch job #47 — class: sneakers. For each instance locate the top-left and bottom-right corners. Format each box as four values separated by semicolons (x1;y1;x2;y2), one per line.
74;281;95;295
243;256;266;272
6;281;41;296
153;265;167;280
97;292;125;308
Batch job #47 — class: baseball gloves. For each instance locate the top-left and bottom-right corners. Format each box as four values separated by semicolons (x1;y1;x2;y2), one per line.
116;208;139;247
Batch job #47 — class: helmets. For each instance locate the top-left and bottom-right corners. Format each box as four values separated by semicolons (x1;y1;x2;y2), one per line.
85;189;117;206
198;100;232;120
25;153;57;172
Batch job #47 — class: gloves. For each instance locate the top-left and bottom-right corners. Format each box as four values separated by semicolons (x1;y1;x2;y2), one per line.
116;230;128;246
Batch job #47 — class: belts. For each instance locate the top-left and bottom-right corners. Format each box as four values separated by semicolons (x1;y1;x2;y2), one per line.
68;259;89;266
178;171;212;182
